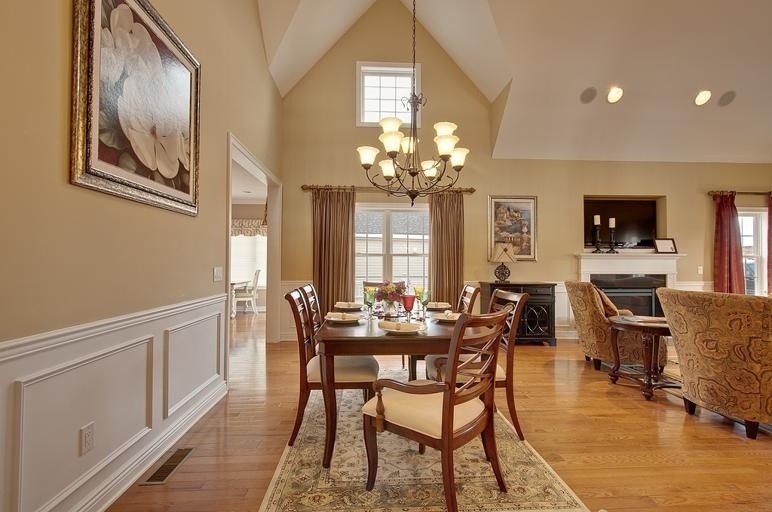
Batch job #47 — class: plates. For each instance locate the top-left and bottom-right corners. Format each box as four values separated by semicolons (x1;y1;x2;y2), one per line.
435;312;463;322
333;303;362;311
323;314;362;325
425;303;449;310
380;322;426;335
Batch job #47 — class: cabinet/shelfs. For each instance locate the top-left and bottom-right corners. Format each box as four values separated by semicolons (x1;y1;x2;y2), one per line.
477;281;558;347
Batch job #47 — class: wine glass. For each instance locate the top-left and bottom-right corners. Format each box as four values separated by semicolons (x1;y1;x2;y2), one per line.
363;286;376;321
399;288;431;324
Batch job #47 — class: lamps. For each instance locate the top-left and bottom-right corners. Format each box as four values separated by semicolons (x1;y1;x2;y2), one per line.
356;0;470;207
490;242;517;283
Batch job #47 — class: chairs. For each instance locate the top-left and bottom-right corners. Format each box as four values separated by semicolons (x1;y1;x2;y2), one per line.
564;281;667;378
655;288;772;440
231;269;261;319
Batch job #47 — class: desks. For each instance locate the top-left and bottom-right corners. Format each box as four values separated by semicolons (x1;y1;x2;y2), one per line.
231;280;251;313
608;316;682;399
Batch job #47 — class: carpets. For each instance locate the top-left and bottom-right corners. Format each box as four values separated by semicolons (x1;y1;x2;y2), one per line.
259;368;590;511
592;353;771;437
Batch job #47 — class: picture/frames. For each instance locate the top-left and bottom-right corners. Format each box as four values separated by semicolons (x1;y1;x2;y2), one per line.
68;1;202;219
653;238;677;253
488;194;536;261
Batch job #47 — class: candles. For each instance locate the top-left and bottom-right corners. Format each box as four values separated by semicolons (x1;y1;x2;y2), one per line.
594;214;616;228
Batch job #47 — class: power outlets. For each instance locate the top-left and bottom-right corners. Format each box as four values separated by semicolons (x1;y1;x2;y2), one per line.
79;421;97;456
